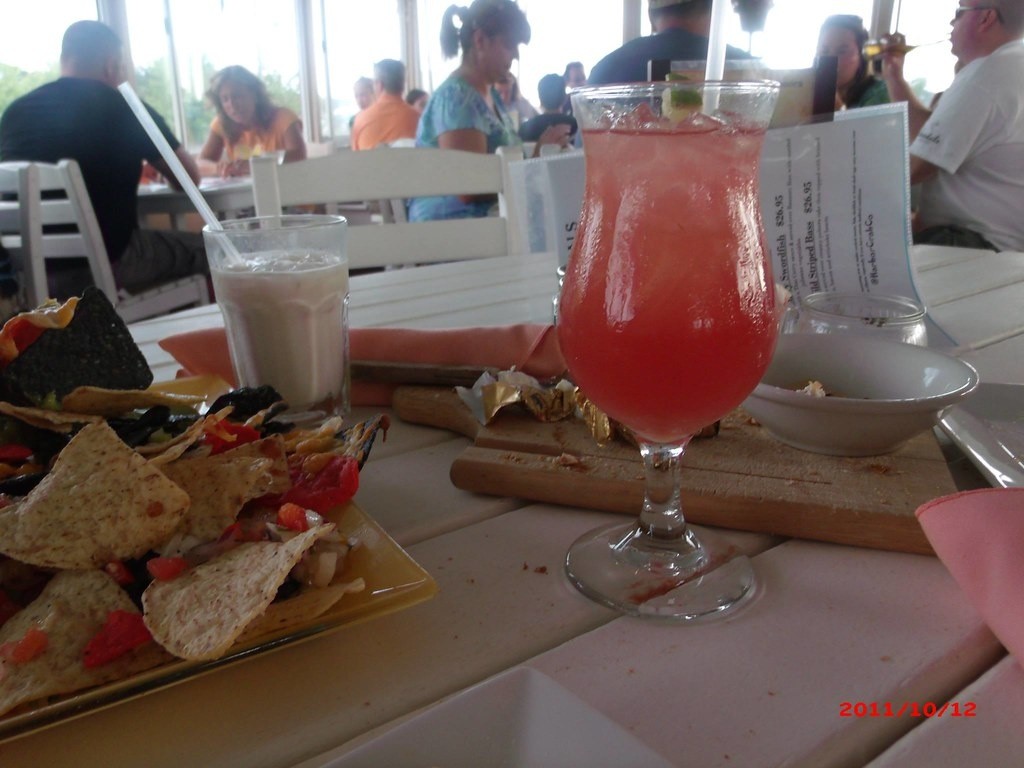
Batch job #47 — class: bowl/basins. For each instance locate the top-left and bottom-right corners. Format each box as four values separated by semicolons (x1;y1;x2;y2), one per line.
739;331;981;456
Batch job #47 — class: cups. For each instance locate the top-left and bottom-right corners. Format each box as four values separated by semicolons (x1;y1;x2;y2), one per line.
202;214;352;419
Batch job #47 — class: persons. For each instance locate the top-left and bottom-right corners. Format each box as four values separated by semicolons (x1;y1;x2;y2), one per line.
589;0;762;88
345;58;430;150
0;20;209;300
815;15;889;111
493;62;586;142
196;65;314;223
884;0;1024;252
407;0;572;268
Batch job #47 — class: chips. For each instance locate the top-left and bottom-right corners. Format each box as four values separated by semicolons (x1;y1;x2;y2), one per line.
0;298;365;717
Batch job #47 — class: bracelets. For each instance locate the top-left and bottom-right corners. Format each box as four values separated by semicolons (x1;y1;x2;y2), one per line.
838;105;847;110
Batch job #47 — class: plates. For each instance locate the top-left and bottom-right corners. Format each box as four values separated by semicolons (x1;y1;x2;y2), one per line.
0;371;439;744
936;381;1024;486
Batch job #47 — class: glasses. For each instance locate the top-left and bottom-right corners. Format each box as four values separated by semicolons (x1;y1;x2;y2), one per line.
955;6;1003;24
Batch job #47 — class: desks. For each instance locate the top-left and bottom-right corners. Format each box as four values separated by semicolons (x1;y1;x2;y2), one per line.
0;246;1024;768
135;176;253;234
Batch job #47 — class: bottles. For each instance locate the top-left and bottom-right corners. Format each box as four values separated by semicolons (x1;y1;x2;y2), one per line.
793;292;929;347
862;31;951;60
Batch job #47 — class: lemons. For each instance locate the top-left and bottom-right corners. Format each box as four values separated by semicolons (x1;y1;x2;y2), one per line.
662;75;703;125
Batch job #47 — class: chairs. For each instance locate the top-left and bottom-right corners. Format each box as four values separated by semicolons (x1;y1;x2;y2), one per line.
250;141;529;283
8;159;210;324
0;162;49;328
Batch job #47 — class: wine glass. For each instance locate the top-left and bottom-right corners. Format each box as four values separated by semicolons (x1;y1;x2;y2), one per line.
554;79;777;623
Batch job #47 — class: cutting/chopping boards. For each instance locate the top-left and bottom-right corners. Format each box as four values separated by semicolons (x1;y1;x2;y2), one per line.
392;384;959;555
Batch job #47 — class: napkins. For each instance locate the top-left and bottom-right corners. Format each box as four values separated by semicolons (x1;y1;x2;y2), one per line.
158;321;562;412
